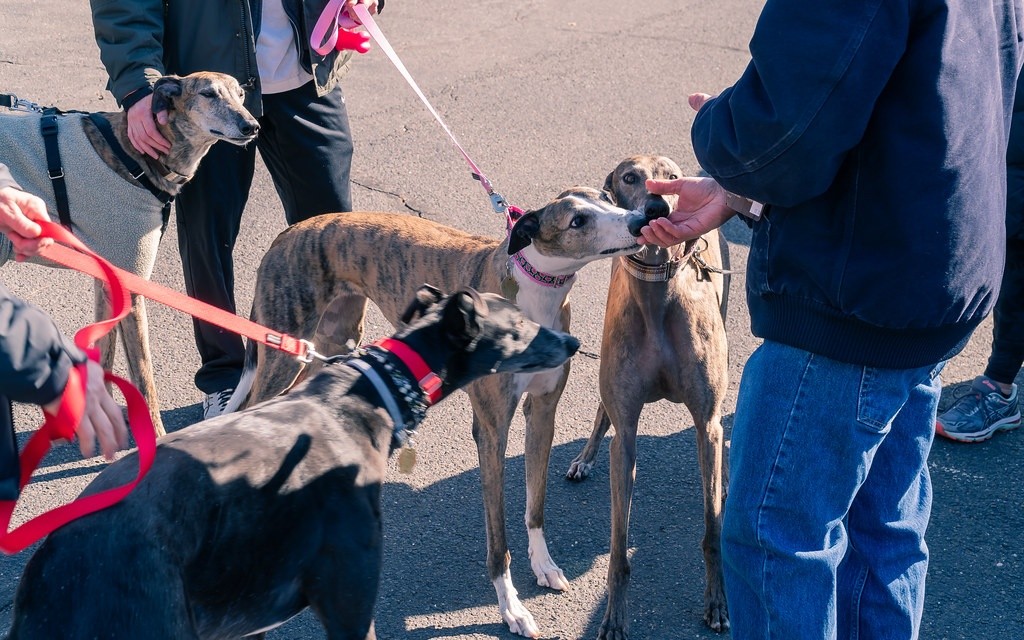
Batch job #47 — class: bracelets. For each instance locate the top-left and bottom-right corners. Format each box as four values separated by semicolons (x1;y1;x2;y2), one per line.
121;85;153;113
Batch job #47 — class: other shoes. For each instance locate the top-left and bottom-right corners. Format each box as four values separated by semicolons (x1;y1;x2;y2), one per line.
203;386;234;421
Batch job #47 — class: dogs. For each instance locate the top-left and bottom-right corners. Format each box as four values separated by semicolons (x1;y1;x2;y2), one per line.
0;283;579;639
565;153;734;639
2;71;262;441
214;187;650;637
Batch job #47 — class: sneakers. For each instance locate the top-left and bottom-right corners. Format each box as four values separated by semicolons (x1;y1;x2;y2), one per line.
935;376;1020;444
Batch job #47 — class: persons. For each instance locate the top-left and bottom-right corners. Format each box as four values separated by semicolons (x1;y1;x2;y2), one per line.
935;62;1024;444
634;0;1024;640
0;163;53;264
0;284;131;501
90;0;386;422
